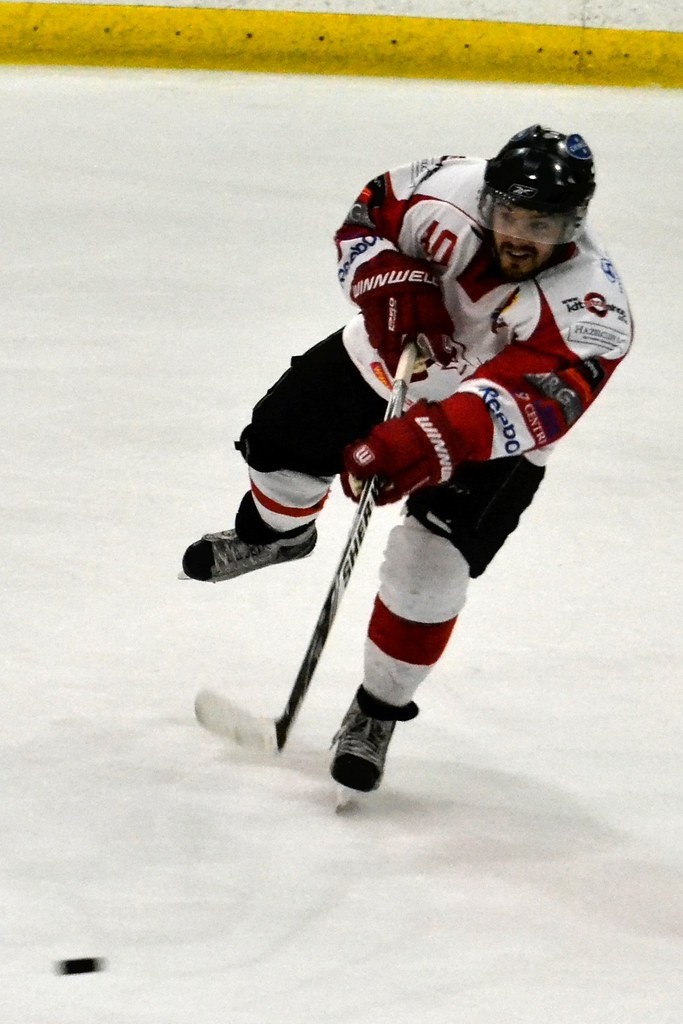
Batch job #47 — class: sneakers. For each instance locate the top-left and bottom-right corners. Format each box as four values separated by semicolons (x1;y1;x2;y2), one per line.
179;489;319;583
328;685;419;813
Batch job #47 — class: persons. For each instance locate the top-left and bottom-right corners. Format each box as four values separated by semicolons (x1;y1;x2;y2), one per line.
179;125;635;813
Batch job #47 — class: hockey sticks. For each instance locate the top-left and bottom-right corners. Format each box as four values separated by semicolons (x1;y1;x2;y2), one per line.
191;336;415;753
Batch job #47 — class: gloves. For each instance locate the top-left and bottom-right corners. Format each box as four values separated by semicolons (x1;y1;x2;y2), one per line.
349;248;456;366
340;398;467;506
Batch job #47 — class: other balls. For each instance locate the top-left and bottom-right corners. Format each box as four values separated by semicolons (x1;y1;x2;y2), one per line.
52;954;102;976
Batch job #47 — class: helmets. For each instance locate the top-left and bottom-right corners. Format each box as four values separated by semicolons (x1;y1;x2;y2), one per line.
485;124;596;231
